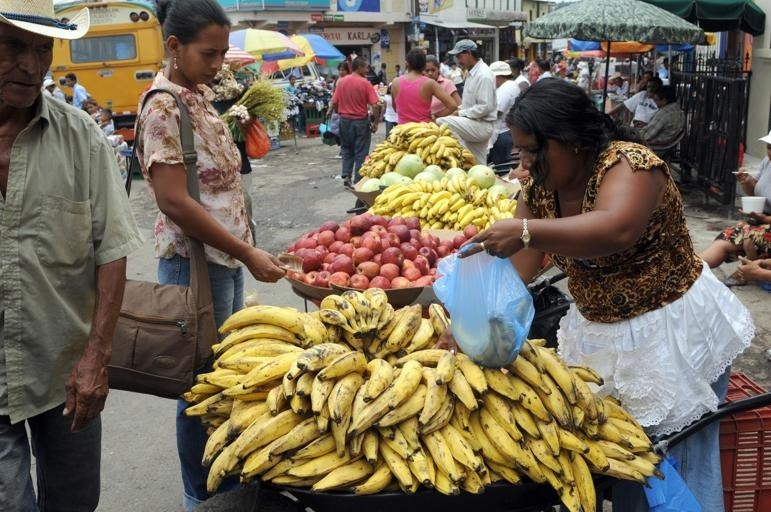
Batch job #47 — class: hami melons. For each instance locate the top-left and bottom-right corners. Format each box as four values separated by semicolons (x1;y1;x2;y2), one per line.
363;155;508;196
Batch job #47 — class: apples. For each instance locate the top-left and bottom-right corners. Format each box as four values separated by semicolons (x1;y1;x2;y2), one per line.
285;213;478;290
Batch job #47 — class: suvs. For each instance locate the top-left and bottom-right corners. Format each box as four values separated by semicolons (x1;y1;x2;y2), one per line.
316;62;380;87
266;60;329;96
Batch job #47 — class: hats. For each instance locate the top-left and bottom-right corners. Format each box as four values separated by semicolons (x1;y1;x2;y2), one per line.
756;130;771;144
489;60;512;76
447;38;478;55
0;2;91;41
43;79;57;88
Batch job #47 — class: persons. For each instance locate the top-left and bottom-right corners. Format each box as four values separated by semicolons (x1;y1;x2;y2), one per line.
1;0;146;512
432;38;498;167
133;0;290;510
43;72;116;138
731;255;771;281
283;49;689;183
458;75;758;511
325;54;381;191
696;128;771;270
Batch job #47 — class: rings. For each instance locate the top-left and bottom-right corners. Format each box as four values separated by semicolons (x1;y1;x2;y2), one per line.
480;242;485;250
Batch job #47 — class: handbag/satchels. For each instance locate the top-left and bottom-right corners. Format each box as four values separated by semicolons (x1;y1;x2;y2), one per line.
527;273;574;348
107;88;219;399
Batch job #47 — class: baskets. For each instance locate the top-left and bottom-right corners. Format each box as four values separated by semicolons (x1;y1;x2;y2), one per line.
717;372;771;511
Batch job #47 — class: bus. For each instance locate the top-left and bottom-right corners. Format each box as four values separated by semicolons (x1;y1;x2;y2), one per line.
45;2;165;179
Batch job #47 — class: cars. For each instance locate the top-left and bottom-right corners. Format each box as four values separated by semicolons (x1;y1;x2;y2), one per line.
598;59;690;90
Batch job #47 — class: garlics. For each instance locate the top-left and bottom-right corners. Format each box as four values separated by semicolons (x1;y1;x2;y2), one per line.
228;104;250;123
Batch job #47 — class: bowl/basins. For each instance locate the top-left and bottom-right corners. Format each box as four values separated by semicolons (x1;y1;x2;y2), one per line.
741;196;767;214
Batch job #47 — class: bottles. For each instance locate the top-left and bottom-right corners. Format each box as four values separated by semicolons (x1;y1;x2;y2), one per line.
379;82;385;97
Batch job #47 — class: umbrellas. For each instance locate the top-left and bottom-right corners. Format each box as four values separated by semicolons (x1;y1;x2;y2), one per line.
258;33;347;74
565;38;696;98
228;27;305;64
520;1;705;110
225;43;256;69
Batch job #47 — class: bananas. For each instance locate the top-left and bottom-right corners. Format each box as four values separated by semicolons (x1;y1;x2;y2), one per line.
368;174;518;231
179;287;664;512
358;121;475;177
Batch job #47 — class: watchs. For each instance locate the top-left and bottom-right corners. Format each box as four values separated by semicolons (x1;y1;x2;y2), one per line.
520;216;532;249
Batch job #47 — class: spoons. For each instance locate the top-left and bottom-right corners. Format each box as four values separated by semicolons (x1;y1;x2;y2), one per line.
733;171;761;176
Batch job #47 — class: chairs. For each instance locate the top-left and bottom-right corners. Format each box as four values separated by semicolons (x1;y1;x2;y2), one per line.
649;131;685;162
